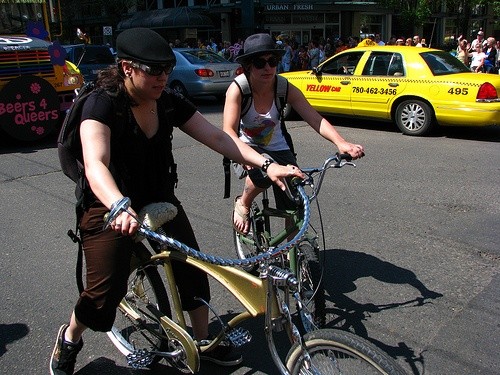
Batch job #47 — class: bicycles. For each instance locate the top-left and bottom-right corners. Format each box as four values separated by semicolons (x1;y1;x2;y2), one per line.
103;164;408;375
233;150;365;334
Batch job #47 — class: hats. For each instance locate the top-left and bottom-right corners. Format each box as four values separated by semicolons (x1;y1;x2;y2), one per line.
117;28;176;65
234;33;286;64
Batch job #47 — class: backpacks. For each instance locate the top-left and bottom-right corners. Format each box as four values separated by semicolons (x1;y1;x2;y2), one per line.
58;81;172;186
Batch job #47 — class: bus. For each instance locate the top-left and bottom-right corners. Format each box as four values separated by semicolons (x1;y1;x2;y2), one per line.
0;0;84;141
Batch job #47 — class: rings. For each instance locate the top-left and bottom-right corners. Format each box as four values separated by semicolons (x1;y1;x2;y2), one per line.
130;221;138;225
116;224;122;227
287;170;293;176
293;166;295;169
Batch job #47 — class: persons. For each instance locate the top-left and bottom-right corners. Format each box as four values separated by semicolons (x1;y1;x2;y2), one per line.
494;40;500;70
471;30;487;52
223;33;364;261
48;27;306;374
367;33;427;47
482;37;497;73
169;35;245;62
456;35;469;68
77;24;94;44
275;31;360;73
465;43;486;73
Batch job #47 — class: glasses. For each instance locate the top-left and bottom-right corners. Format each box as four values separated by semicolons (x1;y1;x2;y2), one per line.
252;56;280;69
131;62;174;76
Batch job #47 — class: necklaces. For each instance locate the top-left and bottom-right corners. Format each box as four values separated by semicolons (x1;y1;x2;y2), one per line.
138;98;156;115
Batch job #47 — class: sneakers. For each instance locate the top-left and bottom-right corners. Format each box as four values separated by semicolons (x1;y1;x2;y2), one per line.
194;335;243;365
49;323;84;375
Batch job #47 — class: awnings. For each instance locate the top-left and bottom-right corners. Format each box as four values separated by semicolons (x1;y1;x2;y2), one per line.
115;6;216;31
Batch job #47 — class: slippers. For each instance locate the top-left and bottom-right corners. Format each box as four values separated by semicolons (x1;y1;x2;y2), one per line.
231;195;251;236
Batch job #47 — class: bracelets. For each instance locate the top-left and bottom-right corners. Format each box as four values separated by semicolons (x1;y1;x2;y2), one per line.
262;158;274;173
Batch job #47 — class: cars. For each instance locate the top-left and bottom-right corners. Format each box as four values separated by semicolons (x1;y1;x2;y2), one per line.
166;48;244;99
60;44;115;82
277;38;500;136
